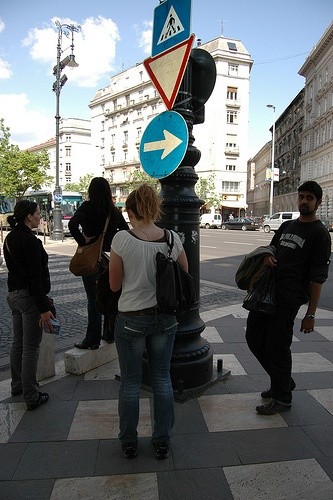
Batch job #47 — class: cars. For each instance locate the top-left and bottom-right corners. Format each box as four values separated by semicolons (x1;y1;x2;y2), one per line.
327;220;333;232
221;218;260;231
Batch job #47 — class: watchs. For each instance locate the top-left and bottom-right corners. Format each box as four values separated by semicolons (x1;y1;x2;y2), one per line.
305;314;315;321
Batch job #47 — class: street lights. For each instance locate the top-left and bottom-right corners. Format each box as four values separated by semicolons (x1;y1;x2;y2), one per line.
52;20;82;240
266;104;276;217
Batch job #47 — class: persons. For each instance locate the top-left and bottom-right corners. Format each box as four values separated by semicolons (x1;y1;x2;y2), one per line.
235;180;331;415
3;200;61;410
68;177;130;349
108;183;197;460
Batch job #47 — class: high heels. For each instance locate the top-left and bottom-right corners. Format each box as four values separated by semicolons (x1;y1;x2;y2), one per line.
101;334;114;343
74;341;99;349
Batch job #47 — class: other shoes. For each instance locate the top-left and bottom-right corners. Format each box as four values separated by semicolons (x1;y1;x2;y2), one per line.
26;391;49;410
122;443;137;457
12;390;23;396
255;400;291;415
155;446;169;459
260;378;296;398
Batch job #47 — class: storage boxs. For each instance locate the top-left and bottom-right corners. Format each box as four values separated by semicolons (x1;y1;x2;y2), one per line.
44;320;61;335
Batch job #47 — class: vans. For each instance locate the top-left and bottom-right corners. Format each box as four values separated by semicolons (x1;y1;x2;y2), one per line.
262;211;300;233
199;213;222;229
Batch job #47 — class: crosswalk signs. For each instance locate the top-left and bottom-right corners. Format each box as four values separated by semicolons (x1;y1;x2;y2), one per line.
151;0;192;58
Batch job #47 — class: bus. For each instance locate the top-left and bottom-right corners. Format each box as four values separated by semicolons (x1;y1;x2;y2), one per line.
0;190;82;237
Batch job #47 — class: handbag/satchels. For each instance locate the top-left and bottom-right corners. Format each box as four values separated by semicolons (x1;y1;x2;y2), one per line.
69;233;105;276
154;229;195;313
45;295;56;318
